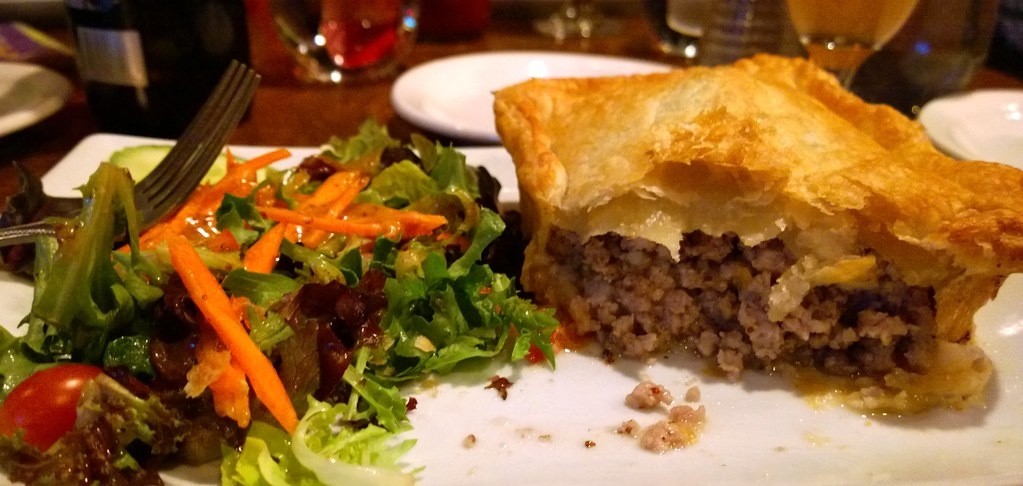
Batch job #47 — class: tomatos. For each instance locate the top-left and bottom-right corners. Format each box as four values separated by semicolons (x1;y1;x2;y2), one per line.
1;363;104;460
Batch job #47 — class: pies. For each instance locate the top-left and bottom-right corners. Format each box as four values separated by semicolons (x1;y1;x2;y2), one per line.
484;49;1023;410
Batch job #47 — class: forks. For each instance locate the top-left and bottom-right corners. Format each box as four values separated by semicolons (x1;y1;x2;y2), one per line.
0;60;262;250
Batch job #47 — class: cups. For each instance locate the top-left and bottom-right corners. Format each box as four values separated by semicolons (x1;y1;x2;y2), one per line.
661;0;803;68
266;0;422;88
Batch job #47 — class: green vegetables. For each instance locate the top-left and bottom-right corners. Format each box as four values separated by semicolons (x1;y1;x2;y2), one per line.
0;115;559;485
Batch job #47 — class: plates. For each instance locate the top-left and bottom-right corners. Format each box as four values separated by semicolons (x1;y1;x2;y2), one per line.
388;48;677;144
1;61;74;139
40;132;320;200
917;87;1023;172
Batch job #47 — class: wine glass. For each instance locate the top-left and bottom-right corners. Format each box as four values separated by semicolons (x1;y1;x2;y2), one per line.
782;0;919;92
